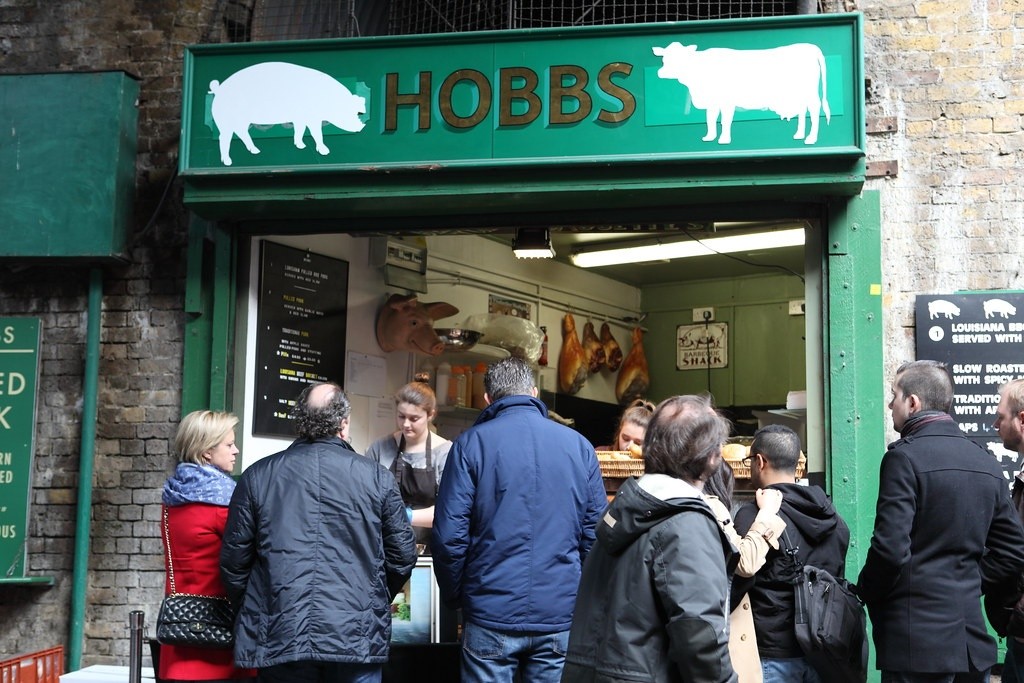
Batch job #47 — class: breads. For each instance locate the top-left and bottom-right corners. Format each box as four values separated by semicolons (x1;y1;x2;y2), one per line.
722;444;751;461
599;444;644;460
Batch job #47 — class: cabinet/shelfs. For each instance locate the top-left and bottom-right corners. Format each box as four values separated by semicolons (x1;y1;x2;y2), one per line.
417;342;512;422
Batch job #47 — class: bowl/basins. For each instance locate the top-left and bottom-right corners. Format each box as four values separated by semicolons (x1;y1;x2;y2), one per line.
432;326;485;351
416;544;426;555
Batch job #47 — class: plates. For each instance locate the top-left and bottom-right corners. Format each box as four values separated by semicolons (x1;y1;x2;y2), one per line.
469;343;511;359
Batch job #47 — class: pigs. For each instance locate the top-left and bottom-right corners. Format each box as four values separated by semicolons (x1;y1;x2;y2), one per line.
377;293;459;357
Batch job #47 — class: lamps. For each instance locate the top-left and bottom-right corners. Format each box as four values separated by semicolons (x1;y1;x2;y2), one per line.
569;221;805;269
513;228;557;260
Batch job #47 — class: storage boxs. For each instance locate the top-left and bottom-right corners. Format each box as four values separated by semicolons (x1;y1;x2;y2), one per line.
0;645;62;683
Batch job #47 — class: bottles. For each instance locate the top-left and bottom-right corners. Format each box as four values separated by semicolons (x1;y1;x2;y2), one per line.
538;326;548;366
447;365;466;408
463;365;472;408
472;361;488;411
435;361;452;407
421;357;435;393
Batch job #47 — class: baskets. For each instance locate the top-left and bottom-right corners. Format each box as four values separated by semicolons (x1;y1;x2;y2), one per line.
594;451;645;477
725;449;806;478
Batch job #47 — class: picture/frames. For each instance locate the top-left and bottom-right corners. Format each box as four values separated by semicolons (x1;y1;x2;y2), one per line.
391;557;441;643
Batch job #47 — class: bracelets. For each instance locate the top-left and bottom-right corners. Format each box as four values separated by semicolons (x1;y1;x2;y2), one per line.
406;507;413;526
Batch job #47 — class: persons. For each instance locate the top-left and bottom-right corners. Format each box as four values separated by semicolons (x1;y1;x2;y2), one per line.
219;384;420;683
157;408;257;683
701;424;852;683
431;359;608;683
983;378;1024;683
854;360;1024;683
363;374;458;554
559;396;743;683
593;398;659;462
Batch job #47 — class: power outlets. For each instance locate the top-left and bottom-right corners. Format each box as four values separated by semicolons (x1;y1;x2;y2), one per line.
692;307;715;322
789;300;805;315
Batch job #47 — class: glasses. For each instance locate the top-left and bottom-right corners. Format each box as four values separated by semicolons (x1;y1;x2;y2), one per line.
742;455;767;467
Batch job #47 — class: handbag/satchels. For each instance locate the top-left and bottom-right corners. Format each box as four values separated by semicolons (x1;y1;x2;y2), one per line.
156;595;236;645
792;565;867;669
